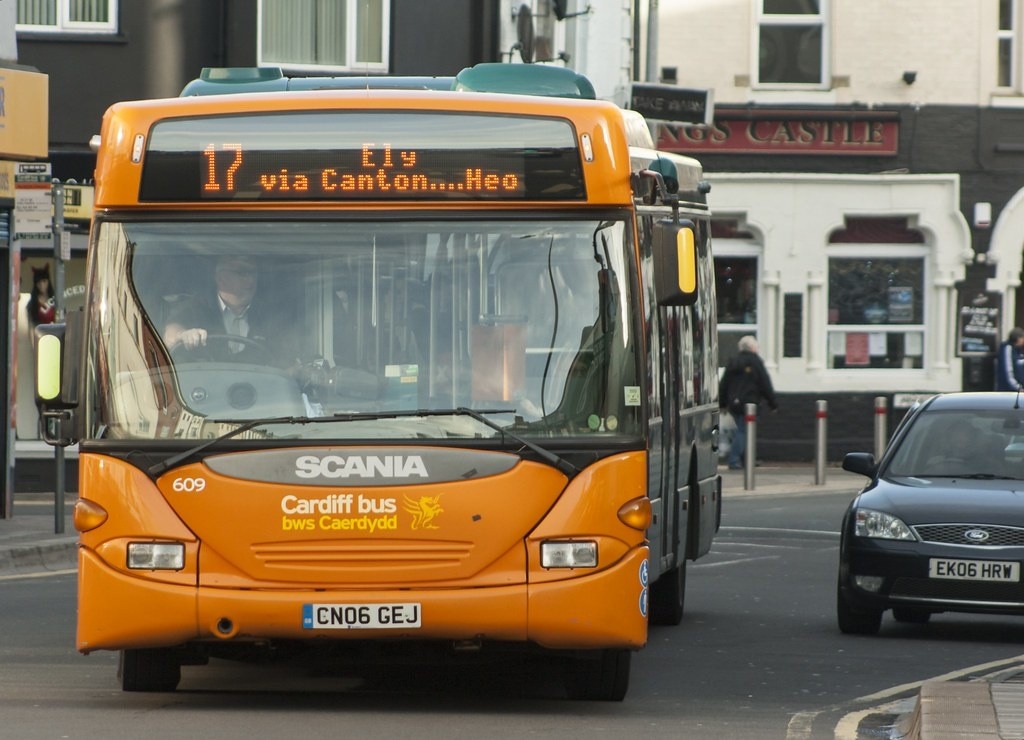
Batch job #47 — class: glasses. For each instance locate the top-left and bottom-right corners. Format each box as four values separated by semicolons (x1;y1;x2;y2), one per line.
219;268;258;277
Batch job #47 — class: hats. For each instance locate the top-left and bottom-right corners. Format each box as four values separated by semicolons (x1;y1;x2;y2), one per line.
32;262;49;283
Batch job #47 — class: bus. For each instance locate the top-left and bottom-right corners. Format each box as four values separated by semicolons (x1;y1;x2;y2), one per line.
34;60;721;707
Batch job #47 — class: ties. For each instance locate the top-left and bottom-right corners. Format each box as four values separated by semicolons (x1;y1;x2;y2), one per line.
230;318;240;352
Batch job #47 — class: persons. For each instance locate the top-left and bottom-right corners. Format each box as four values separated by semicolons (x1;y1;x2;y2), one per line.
158;250;299;373
27;263;67;439
715;335;781;469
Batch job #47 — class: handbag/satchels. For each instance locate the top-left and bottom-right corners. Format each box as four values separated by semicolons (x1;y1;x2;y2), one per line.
719;411;738;457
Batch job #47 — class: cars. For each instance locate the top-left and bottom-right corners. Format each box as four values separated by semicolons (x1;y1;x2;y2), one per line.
837;392;1024;635
